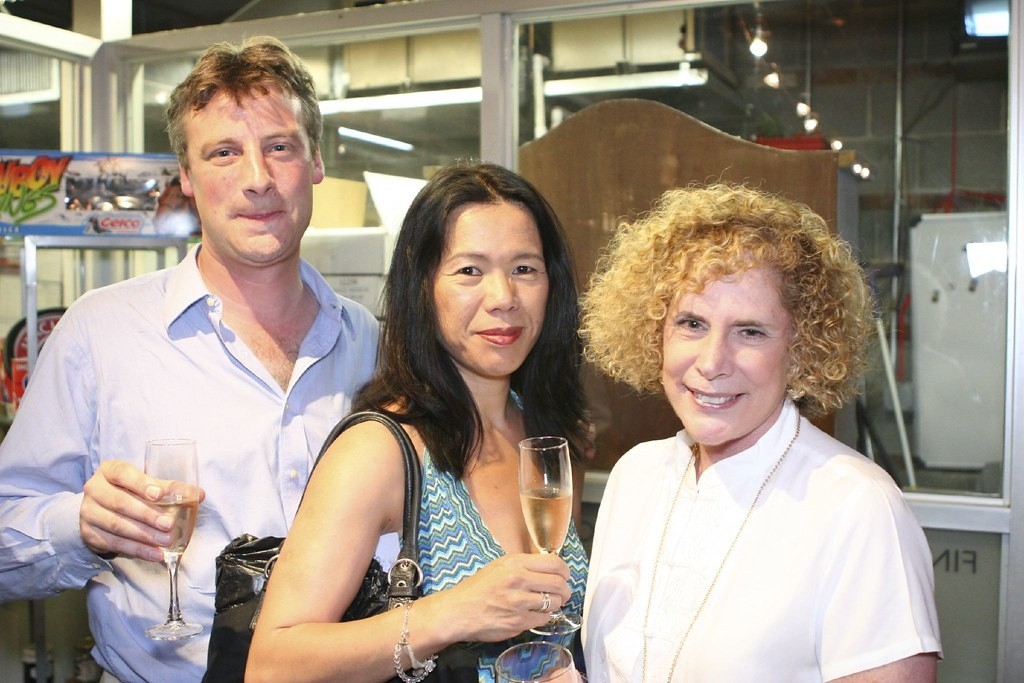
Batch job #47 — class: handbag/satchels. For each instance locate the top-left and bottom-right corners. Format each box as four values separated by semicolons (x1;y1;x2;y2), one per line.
201;412;477;683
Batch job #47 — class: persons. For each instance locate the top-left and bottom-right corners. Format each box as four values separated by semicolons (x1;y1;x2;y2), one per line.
242;160;591;683
578;184;946;683
1;34;387;683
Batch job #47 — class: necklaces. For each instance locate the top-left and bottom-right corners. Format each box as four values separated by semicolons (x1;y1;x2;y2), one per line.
638;402;804;681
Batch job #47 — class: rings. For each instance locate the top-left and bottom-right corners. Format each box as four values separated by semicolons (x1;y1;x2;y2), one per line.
536;590;552;614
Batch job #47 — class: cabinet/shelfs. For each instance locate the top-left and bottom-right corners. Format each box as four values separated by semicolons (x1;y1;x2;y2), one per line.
19;234;189;683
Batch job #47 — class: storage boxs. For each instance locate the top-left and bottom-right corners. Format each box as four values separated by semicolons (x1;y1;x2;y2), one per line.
322;276;387;319
300;226;398;277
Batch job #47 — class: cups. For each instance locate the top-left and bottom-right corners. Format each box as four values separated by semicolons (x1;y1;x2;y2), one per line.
495;641;580;683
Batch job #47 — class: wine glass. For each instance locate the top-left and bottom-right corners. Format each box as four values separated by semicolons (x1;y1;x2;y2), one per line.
518;437;584;635
143;438;204;640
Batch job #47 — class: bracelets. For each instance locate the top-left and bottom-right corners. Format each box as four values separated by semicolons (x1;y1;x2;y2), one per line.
392;595;441;682
